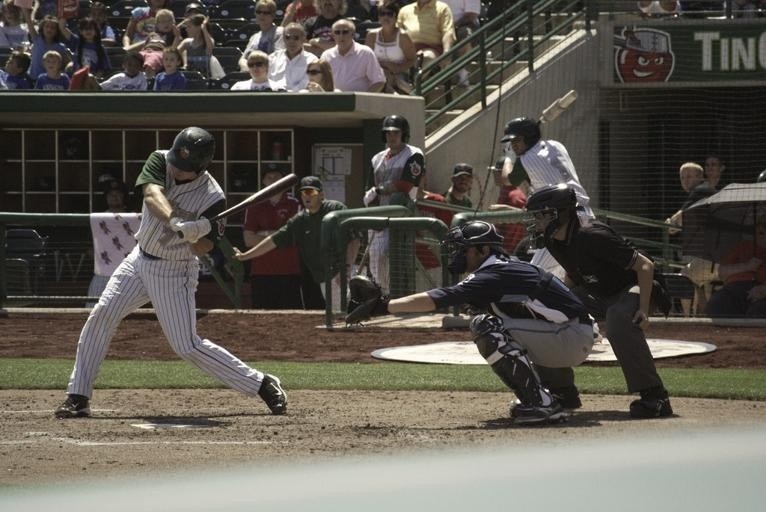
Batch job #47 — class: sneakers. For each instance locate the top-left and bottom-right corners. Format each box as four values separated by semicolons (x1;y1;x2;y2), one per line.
54;392;91;419
507;388;583;425
256;373;288;414
458;69;469;87
629;388;672;419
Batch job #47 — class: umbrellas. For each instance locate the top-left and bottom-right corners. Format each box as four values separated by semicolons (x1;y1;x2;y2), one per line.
682;179;766;282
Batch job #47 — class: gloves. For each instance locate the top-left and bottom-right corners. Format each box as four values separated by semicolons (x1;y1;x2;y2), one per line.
363;187;378;208
170;216;211;243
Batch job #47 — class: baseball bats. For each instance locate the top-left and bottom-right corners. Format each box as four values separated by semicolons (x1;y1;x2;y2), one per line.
537;90;578;125
177;173;297;238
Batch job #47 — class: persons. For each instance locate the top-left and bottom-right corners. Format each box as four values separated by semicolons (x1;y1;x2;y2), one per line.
702;151;728;192
634;0;766;21
50;124;292;421
702;216;766;318
1;1;520;91
522;182;673;419
341;218;598;424
665;160;719;316
86;114;600;319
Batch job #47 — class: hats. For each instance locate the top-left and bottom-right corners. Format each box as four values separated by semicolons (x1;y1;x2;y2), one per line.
453;164;473;178
487;157;505;172
298;175;323;192
184;2;204;14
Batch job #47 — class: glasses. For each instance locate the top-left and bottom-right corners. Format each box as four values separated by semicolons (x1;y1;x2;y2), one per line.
247;10;395;75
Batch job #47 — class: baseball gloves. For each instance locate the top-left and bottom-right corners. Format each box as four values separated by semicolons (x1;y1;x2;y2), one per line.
343;272;390;325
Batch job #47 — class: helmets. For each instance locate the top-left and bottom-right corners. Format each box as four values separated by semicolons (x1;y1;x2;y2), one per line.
446;218;505;273
523;182;580;249
381;114;409;143
166;126;216;172
499;116;541;144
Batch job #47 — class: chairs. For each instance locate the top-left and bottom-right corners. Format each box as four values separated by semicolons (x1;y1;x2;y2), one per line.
1;254;34;298
1;225;54;296
